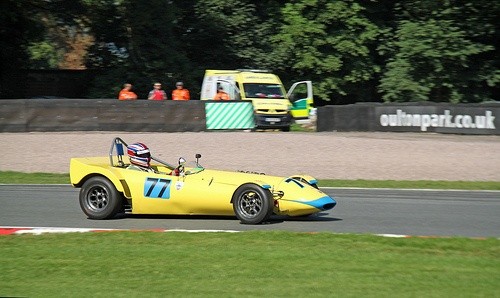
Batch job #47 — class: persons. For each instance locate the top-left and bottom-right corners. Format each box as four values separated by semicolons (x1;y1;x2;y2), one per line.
118;83;138;100
214;87;230;100
148;83;167;100
126;142;179;176
172;82;190;100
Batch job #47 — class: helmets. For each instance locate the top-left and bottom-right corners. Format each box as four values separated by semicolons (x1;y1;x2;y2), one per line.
127;143;152;169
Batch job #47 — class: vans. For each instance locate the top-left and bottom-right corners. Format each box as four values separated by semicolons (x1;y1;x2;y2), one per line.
200;68;314;132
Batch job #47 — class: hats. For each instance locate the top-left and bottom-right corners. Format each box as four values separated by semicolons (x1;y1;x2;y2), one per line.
154;83;161;85
176;82;183;86
